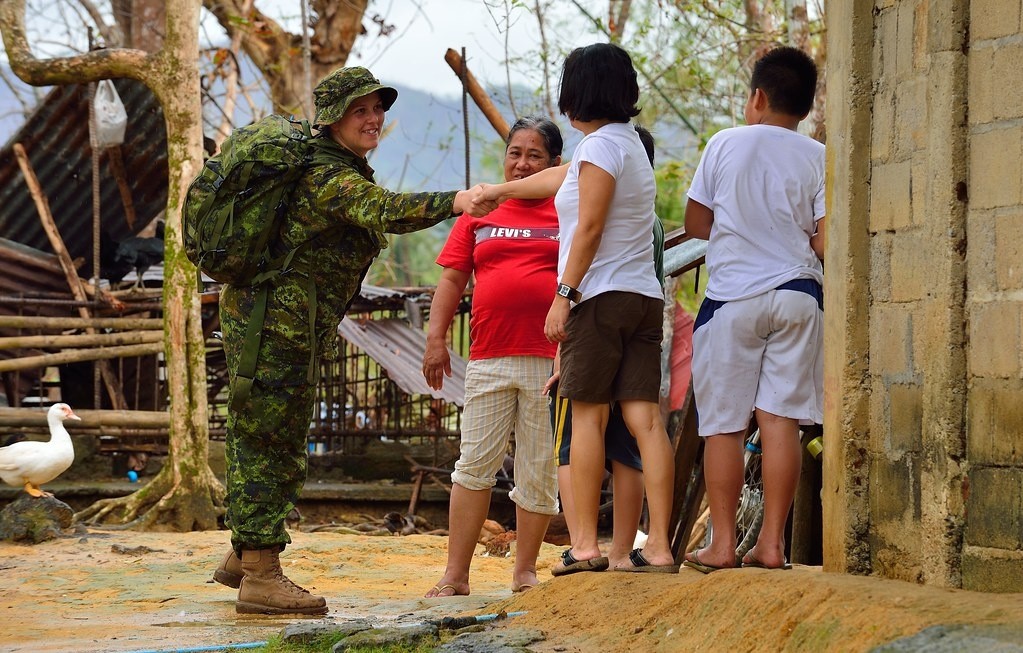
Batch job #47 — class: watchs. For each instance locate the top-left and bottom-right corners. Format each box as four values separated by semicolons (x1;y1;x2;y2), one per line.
555;282;583;302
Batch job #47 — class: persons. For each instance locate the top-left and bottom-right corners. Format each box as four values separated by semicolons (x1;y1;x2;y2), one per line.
684;46;826;572
213;66;498;617
421;115;563;599
471;42;684;577
544;126;663;565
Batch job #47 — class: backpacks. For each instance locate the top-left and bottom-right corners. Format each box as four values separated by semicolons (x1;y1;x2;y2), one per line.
182;114;312;288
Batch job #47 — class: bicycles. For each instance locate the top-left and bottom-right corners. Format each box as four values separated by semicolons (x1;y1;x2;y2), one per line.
702;409;802;569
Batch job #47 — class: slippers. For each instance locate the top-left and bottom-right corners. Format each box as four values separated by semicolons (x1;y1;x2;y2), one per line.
614;548;680;573
424;585;471;600
551;548;609;576
742;548;792;570
683;549;717;573
512;584;534;596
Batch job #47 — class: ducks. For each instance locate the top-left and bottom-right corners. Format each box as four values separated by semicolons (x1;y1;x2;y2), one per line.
0;403;82;497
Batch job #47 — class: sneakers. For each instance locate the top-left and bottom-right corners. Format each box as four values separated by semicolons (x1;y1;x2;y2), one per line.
236;546;329;615
213;547;245;588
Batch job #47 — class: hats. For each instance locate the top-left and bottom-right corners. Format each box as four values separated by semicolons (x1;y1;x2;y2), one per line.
312;65;398;130
